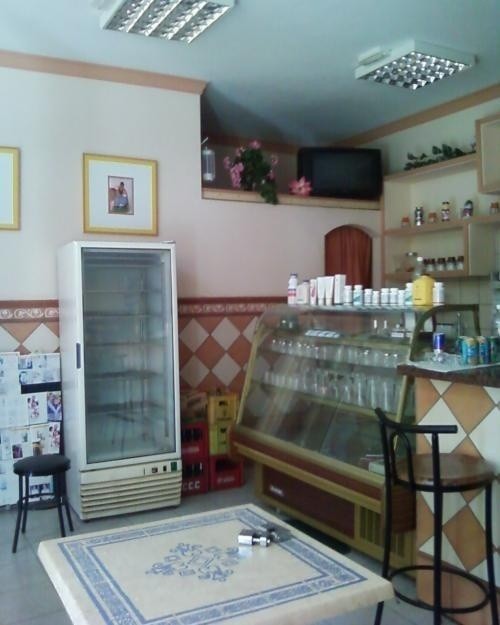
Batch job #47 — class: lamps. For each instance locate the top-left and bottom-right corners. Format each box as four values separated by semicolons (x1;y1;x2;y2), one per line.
356;38;476;90
98;0;236;44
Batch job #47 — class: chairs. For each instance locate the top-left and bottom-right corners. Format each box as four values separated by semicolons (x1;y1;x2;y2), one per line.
374;405;500;625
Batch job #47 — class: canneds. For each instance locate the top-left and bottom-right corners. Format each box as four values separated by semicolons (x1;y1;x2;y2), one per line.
454;334;499;364
433;332;446;355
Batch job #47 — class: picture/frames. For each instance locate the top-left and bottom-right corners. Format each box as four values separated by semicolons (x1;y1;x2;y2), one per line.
82;152;158;236
0;146;20;231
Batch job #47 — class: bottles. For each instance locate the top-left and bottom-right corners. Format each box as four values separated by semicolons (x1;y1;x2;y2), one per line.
269;338;402;369
491;304;500;335
288;271;299;305
411;255;444;307
456;311;464;340
344;284;411;307
299;279;311;304
441;201;450;221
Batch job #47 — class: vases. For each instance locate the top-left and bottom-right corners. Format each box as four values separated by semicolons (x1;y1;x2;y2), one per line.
244;173;252;190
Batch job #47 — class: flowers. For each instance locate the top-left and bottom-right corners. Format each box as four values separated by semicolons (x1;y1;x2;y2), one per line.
287;177;312;195
224;139;280;206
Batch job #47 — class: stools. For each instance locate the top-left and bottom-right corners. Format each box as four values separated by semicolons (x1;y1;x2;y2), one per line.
11;455;73;553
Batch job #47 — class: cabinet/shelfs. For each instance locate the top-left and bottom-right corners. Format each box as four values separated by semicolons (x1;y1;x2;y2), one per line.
379;113;500;287
228;304;480;581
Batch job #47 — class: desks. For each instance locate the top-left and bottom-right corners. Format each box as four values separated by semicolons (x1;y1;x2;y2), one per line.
38;504;394;625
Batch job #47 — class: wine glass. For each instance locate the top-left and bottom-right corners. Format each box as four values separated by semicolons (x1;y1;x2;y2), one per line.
265;367;354;403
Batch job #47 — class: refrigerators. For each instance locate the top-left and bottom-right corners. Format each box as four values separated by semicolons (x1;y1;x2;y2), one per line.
58;240;182;520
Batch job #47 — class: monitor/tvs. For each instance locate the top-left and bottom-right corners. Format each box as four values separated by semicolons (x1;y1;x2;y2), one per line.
297;148;382;200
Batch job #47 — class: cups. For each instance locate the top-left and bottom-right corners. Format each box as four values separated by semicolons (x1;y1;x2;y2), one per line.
423;351;463;372
354;374;402;414
414;206;423;225
